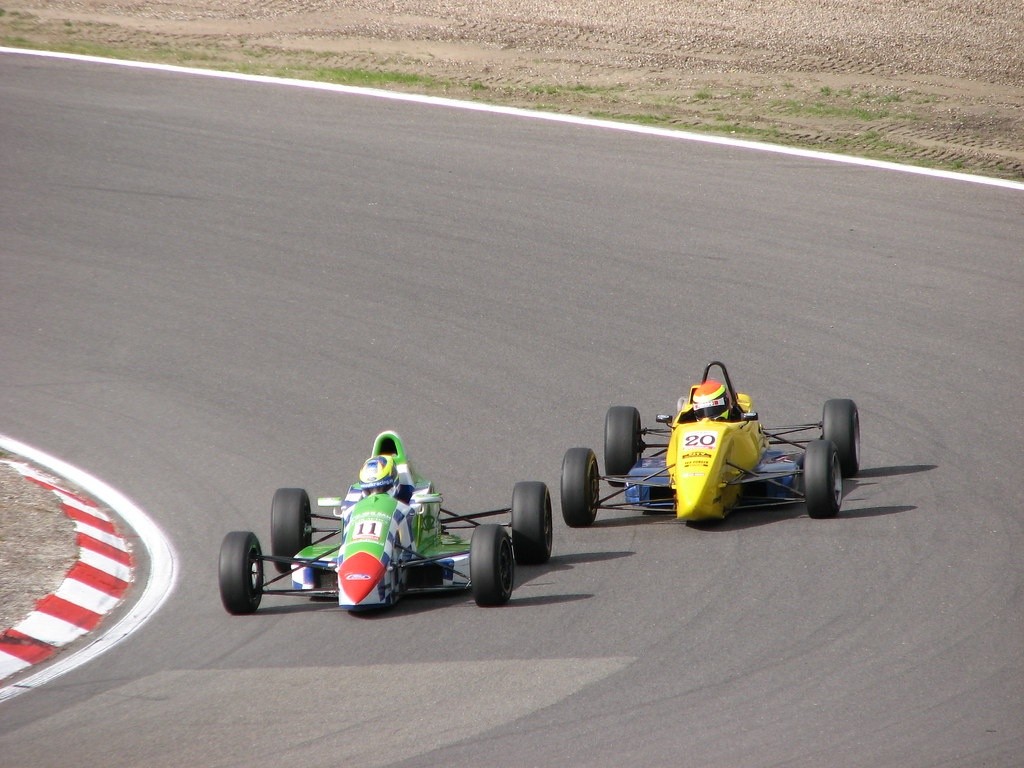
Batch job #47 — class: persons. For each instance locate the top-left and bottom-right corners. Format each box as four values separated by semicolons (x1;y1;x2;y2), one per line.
358;454;415;506
679;380;742;423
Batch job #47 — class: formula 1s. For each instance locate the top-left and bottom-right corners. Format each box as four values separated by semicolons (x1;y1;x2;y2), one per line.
560;363;864;524
217;428;551;615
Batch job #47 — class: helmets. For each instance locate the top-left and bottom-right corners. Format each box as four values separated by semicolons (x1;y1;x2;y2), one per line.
358;455;400;497
691;382;729;422
